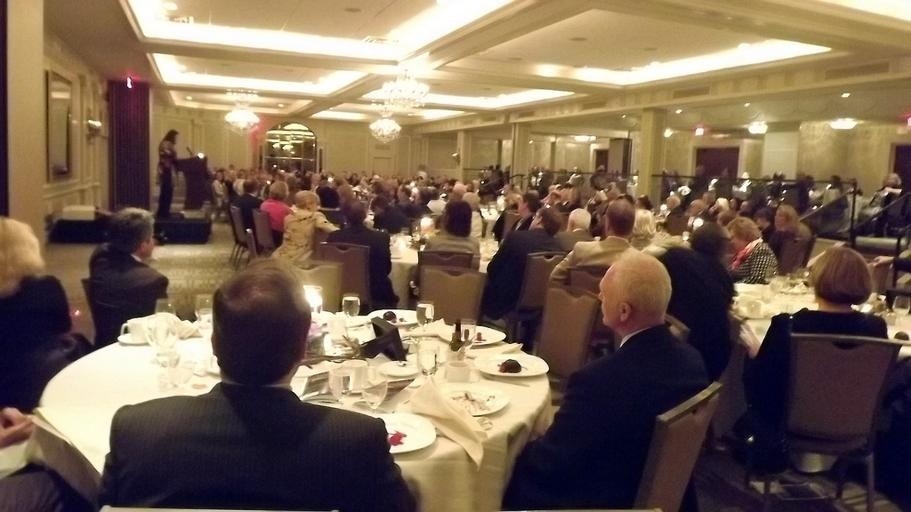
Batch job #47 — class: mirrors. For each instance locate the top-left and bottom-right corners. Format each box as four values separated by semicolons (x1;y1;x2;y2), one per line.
46;66;75;185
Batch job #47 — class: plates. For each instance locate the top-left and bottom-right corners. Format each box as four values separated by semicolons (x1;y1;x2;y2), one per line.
732;277;886;319
387;253;402;259
886;330;911;346
118;313;549;456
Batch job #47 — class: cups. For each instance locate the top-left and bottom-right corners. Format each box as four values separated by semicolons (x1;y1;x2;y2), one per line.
341;293;361;316
155;299;177;317
302;286;322;315
417;299;435;325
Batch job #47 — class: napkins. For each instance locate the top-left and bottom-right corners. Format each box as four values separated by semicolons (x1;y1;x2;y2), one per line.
405;382;488;471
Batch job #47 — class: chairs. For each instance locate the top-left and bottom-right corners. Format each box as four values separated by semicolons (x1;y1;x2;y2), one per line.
632;381;724;512
767;184;911;306
744;332;904;512
208;170;693;380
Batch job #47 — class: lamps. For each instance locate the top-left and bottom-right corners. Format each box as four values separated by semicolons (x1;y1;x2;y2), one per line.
217;101;263;131
379;72;432;114
366;116;406;146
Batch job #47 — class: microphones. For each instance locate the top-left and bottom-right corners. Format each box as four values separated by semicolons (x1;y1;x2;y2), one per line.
187;146;193;154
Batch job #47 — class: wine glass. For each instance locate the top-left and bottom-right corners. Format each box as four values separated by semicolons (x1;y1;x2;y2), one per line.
379;226;421;242
484;231;495;247
194;295;214;330
892;296;910;326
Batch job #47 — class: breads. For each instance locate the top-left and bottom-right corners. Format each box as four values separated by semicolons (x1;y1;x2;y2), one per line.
499;359;521;373
